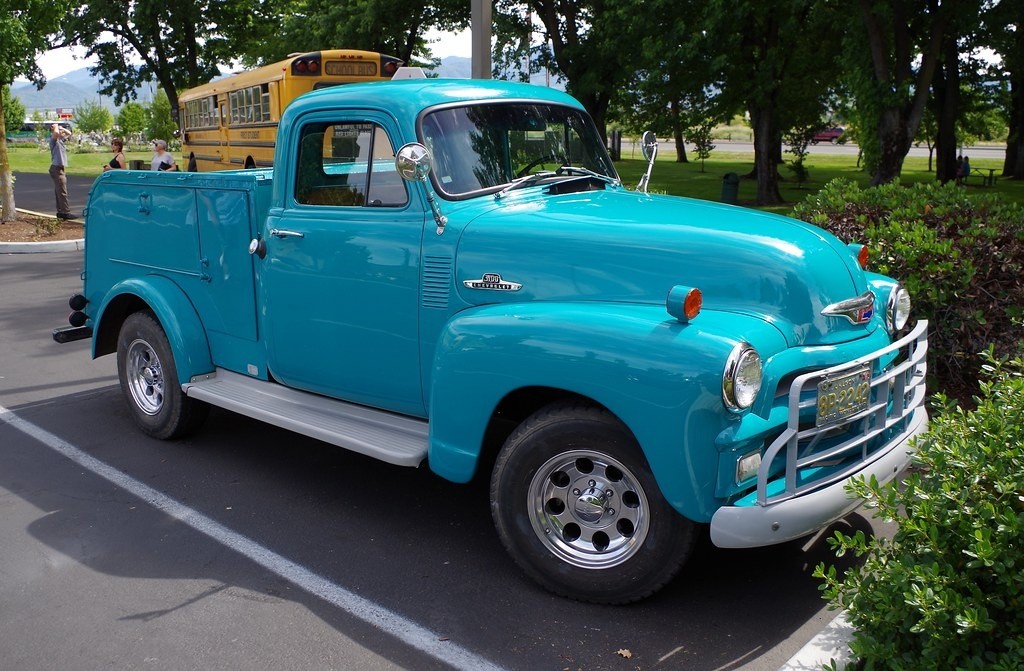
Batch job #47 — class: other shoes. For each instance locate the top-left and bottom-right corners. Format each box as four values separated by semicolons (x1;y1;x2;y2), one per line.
56;213;63;218
62;213;78;219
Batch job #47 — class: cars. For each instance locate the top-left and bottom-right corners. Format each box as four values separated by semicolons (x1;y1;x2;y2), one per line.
811;127;849;145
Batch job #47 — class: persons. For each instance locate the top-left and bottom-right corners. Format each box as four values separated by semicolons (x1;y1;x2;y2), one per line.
953;155;970;185
151;140;176;171
103;139;126;171
48;123;79;221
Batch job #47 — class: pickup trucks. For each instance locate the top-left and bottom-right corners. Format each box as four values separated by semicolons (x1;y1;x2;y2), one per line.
53;67;929;605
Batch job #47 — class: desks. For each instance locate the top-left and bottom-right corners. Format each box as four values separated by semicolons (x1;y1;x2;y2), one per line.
969;167;1003;187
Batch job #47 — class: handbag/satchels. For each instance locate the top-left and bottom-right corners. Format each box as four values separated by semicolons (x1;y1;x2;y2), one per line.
157;161;178;171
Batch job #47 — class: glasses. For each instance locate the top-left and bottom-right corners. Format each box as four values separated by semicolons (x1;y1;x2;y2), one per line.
152;141;166;148
110;143;117;146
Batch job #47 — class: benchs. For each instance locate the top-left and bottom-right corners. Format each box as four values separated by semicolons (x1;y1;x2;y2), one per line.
967;175;999;185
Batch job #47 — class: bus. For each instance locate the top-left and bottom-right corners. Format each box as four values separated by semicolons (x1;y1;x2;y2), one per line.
5;121;70;143
171;50;407;172
